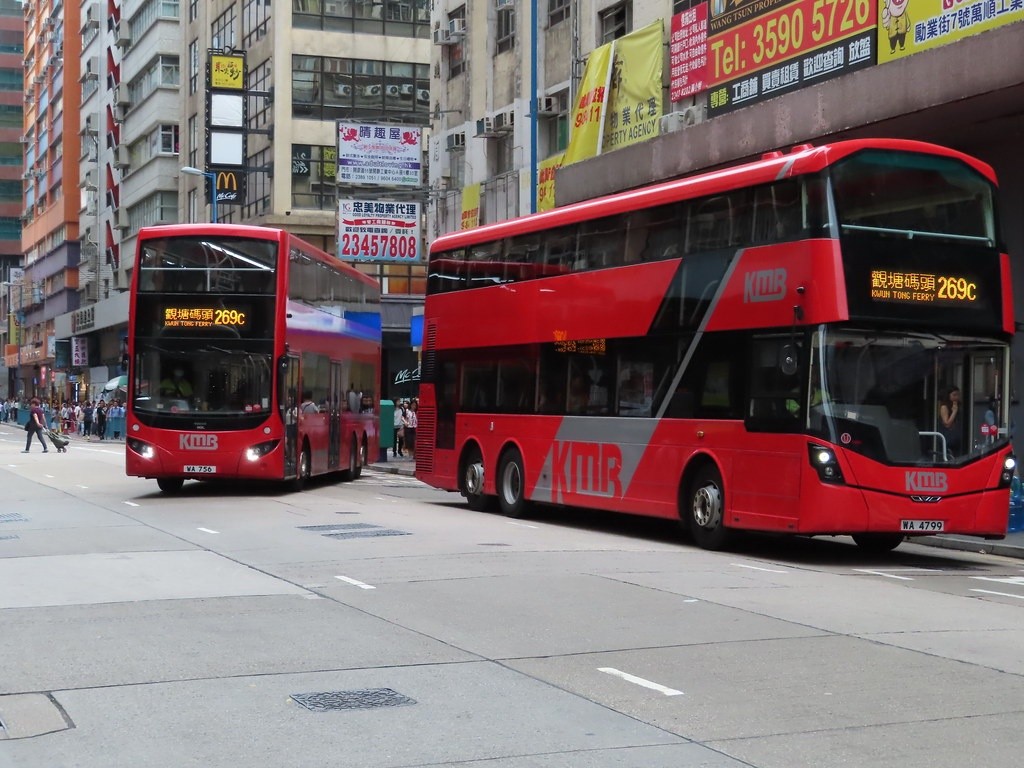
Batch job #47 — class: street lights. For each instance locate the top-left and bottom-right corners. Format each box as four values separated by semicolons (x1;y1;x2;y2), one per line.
181;166;218;223
3;282;24;395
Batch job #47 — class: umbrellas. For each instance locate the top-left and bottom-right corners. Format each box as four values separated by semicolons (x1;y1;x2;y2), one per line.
102;374;139;393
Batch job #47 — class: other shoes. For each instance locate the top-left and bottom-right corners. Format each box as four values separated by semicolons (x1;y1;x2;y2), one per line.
393;452;397;457
398;451;403;458
42;449;49;453
87;436;90;441
21;450;30;453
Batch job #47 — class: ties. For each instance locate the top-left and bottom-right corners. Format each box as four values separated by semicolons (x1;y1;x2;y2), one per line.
175;384;179;396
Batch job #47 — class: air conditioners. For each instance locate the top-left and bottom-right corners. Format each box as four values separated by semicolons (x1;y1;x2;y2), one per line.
497;0;515;7
449;18;464;35
417;89;430;101
401;84;412;95
335;85;352;96
477;118;492;134
433;29;453;45
530;96;559;114
366;85;382;96
495;112;511;127
448;134;464;146
385;85;398;96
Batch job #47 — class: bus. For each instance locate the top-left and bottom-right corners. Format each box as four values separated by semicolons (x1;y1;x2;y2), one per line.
120;222;383;492
412;135;1023;555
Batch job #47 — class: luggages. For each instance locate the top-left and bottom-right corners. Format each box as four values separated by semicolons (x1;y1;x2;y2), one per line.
42;425;70;453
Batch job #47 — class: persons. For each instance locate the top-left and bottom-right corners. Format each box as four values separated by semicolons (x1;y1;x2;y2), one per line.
158;368;192;398
784;372;830;416
21;398;48;452
0;395;126;440
391;396;418;457
285;396;299;463
303;383;374;414
940;384;964;457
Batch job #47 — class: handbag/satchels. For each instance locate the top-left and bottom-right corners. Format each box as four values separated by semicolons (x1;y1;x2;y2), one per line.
397;428;404;437
24;420;31;431
